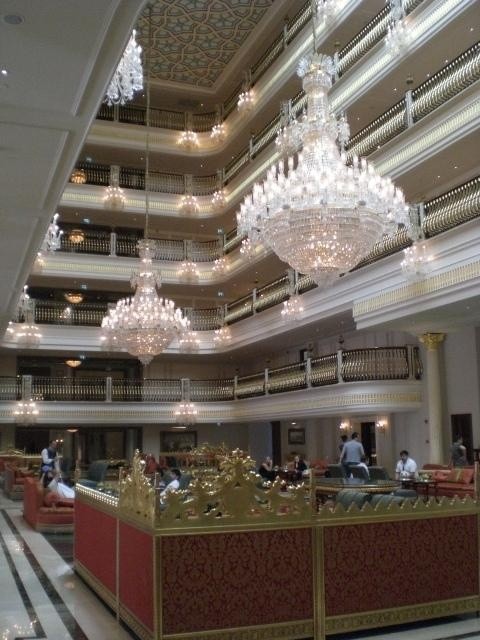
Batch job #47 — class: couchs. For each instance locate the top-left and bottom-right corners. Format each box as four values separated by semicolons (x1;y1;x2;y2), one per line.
3;462;75;535
413;462;476;500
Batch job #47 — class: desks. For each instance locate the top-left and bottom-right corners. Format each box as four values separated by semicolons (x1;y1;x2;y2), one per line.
303;478;401;514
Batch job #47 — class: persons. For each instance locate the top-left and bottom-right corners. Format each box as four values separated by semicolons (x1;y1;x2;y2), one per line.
45;470;75;498
339;432;367;480
160;468;181;511
259;456;274;482
447;433;470;467
395;450;419;481
339;435;347;451
293;456;307;485
40;439;58;487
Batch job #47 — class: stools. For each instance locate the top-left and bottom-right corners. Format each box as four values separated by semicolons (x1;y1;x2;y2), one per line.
78;462;108;490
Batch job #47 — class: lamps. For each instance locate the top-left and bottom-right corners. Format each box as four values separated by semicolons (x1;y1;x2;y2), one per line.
232;1;411;292
96;0;193;370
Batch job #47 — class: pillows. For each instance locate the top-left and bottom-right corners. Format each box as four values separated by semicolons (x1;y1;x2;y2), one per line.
447;467;475;484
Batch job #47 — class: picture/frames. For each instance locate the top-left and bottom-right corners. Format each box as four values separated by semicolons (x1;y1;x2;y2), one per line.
159;430;197;452
288;428;306;444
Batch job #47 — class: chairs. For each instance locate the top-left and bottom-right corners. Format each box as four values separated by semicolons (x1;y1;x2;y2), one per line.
156;473;191;512
326;464;389;481
